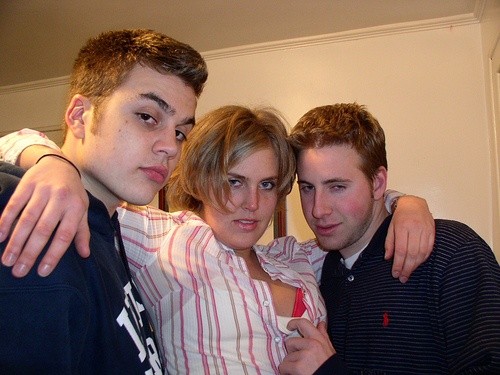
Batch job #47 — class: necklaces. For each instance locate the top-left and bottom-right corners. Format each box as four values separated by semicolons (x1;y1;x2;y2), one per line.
244;253;255;259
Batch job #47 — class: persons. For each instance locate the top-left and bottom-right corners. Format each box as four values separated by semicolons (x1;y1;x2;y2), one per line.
0;106;435;375
288;102;500;375
0;30;208;375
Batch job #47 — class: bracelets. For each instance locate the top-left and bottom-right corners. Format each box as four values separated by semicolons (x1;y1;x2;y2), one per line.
36;154;81;179
395;199;398;209
